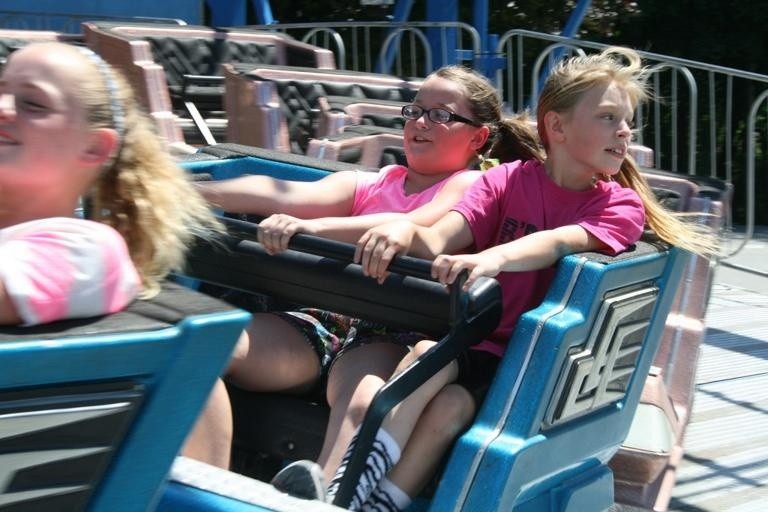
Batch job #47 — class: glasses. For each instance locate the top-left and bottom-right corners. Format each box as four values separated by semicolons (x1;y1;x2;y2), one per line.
401;104;482;130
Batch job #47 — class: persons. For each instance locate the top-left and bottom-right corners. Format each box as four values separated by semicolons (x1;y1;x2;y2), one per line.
325;45;717;512
0;39;229;331
178;63;540;498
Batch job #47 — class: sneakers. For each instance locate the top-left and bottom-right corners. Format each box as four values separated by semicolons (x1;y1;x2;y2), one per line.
270;459;327;504
